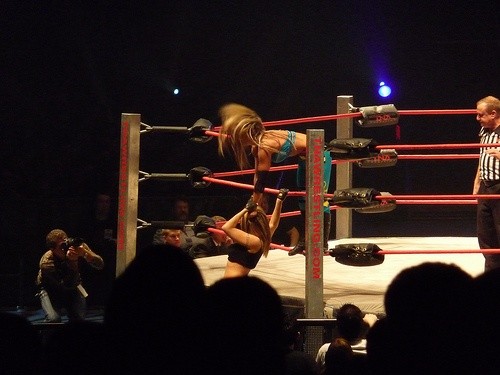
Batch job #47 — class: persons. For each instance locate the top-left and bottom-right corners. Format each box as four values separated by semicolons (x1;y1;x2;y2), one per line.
195;189;287;279
473;96;500;272
140;199;320;243
36;229;104;322
77;191;121;255
0;246;500;375
184;102;332;257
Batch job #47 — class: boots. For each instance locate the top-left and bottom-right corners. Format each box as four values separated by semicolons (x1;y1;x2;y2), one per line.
288;210;306;256
323;212;331;251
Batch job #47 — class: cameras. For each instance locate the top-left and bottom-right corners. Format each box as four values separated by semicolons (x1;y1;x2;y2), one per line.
60;237;83;254
